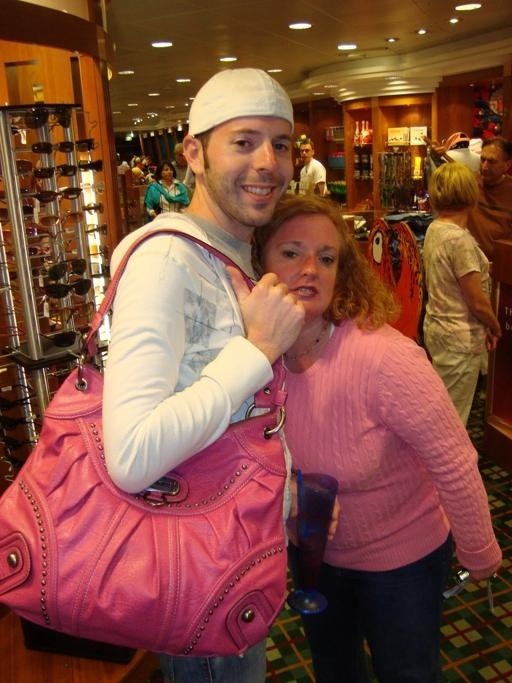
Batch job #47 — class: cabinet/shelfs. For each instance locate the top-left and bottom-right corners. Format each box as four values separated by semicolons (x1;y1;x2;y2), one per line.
117;173;153;238
311;95;438;265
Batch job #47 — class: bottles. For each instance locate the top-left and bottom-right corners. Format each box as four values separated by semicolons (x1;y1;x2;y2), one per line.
325;119;374;201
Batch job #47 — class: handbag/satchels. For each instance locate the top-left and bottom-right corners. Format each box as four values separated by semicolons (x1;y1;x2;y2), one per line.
0;226;295;658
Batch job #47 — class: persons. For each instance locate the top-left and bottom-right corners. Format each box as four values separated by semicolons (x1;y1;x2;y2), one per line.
422;133;511;261
142;161;190;224
251;189;502;682
298;138;328;197
420;161;500;427
115;142;195;208
107;67;304;683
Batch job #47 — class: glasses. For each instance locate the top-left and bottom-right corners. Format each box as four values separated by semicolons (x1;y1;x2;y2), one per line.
0;397;32;469
12;106;111;348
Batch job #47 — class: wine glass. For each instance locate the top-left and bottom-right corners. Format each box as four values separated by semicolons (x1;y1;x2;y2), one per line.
286;473;339;615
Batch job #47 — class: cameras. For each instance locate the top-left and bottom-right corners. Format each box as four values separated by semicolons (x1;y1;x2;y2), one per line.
448;569;471;585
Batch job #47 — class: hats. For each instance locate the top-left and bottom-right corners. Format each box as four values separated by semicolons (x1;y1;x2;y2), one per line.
186;64;300;138
